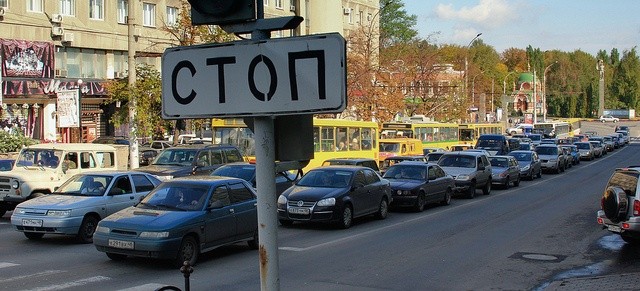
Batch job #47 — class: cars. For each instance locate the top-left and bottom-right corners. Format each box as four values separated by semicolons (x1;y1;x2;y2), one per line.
437;151;492;199
561;147;572;168
474;134;505;154
508;125;533;132
533;132;542;143
89;176;270;268
512;149;541;181
276;165;393;228
512;133;521;150
150;140;174;152
428;147;438;163
490;150;521;189
562;144;580;165
323;155;383;172
10;172;156;240
523;137;533;147
538;143;565;176
522;127;531;138
590;122;628;155
212;162;303;197
387;155;424;166
450;144;473;151
573;140;594;161
137;148;158;165
386;161;458;211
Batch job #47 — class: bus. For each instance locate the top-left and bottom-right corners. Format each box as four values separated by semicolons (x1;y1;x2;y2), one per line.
542;116;583;137
379;121;458;161
210;118;382;189
460;122;505;148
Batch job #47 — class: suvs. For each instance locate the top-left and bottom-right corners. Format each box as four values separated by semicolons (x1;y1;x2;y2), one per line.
595;164;640;241
599;114;621;124
137;143;242;177
94;135;141;144
0;142;131;229
383;137;424;164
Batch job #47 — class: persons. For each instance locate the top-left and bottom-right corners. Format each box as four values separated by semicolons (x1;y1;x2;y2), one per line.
331;130;375;150
40;154;48;163
418;130;458;142
175;155;181;163
112;180;125;194
82;177;101;194
475;128;500;138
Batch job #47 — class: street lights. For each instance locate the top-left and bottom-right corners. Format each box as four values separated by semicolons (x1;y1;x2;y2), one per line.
463;33;483;124
368;0;395;34
503;70;519;94
471;69;489;107
543;60;559;122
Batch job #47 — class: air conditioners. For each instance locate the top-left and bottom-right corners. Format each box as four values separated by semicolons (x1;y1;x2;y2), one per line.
0;7;6;17
52;14;63;23
52;26;64;36
62;32;73;42
